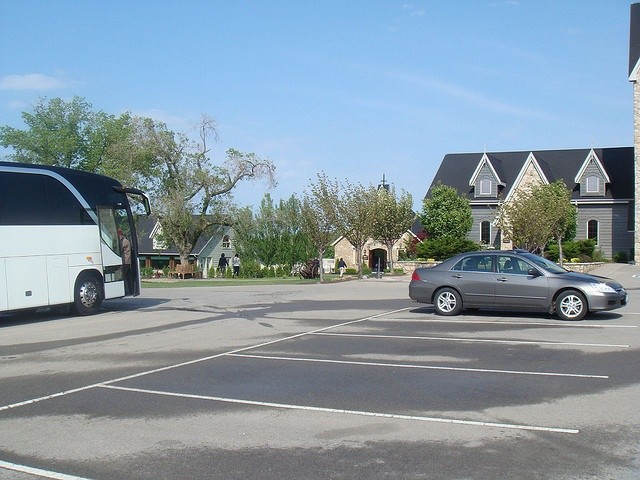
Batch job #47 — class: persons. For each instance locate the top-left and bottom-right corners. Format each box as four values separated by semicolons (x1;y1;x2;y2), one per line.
218;252;229;278
337;257;348;278
117;228;132;295
232;254;243;279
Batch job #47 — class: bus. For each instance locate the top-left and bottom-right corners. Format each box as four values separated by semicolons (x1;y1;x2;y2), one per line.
0;161;150;316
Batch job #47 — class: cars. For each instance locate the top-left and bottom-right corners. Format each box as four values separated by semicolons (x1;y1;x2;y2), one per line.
409;249;628;320
